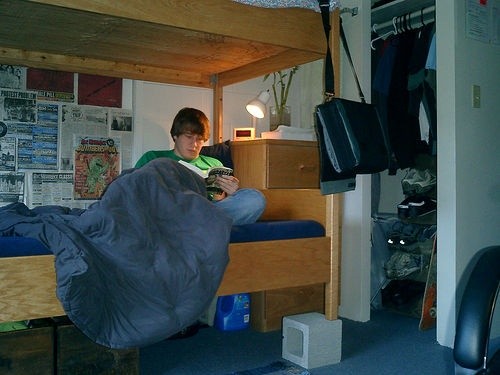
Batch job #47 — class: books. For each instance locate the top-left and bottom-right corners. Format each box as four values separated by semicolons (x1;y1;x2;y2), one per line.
179;160;233;195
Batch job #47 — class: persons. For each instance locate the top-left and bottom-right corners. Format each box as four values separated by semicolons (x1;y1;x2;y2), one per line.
134;107;265;225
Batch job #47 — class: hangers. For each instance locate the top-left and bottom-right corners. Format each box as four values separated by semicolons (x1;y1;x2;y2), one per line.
370;8;433;44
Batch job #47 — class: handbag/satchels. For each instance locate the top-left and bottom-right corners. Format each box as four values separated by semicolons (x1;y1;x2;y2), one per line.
314;97;390;195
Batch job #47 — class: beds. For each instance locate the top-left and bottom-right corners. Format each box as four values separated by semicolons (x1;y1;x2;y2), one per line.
0;0;342;324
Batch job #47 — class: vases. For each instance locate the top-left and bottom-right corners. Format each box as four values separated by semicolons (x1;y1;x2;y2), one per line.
270;106;291;130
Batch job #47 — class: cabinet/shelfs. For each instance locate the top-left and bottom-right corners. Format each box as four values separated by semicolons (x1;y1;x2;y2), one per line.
375;212;435;331
224;139;327;334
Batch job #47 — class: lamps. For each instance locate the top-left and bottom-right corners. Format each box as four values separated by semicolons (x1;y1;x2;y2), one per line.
246;89;271;128
